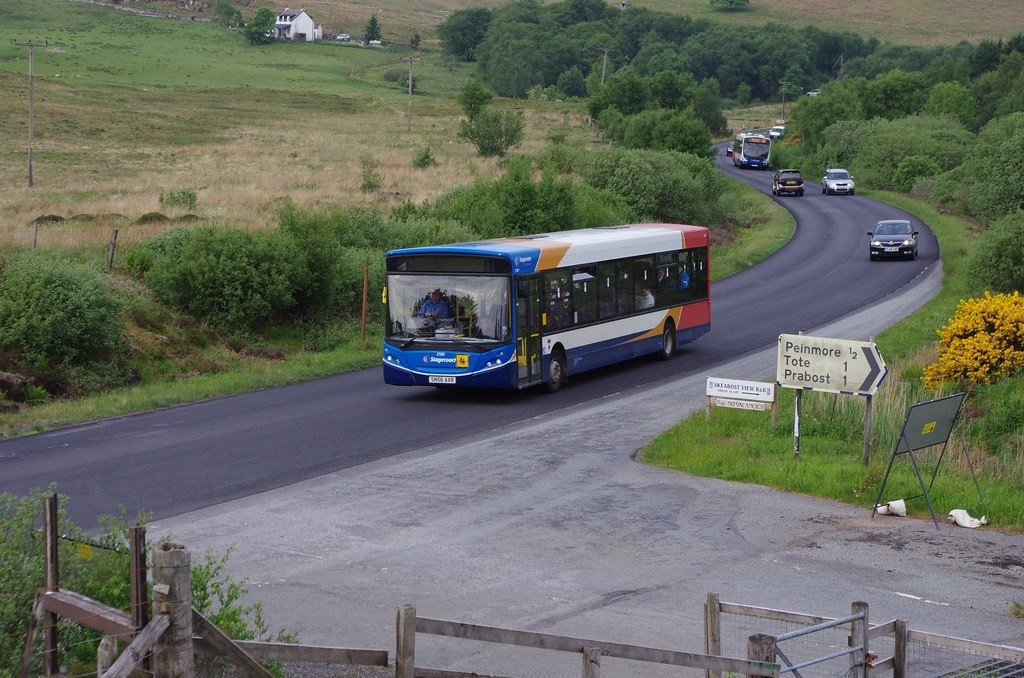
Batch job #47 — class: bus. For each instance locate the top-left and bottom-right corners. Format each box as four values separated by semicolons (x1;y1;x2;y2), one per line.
381;224;712;395
732;133;772;170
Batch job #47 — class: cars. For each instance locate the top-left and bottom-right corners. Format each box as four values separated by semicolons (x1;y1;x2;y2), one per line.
867;219;920;261
807;89;822;97
727;142;735;156
336;34;350;42
769;126;785;139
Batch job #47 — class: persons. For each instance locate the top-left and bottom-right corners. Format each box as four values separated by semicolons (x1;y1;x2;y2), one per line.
546;264;690;329
417;287;449;324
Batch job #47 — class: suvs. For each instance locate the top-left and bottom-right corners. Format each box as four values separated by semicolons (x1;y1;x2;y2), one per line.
822;168;855;195
771;169;805;197
